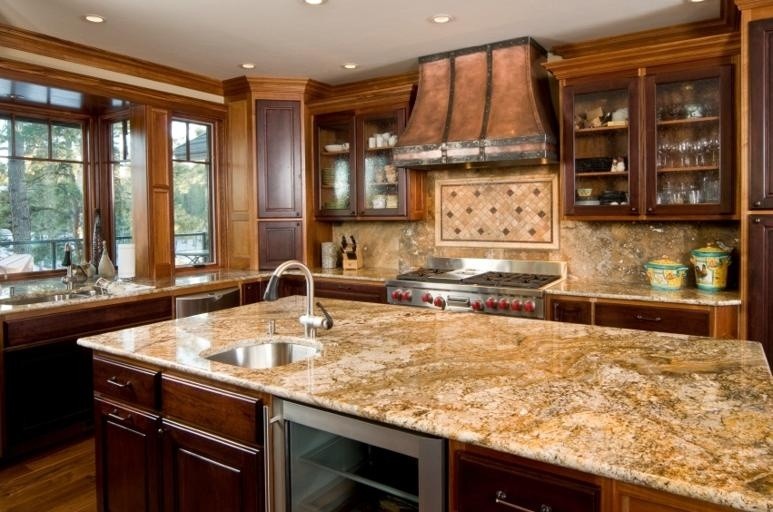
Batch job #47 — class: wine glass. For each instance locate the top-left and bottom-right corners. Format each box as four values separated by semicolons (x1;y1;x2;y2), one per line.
656;136;719;167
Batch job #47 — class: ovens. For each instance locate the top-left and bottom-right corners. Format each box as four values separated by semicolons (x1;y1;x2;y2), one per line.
174;288;240;318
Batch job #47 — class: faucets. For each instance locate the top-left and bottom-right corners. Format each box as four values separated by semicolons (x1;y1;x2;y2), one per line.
60;241;75;290
261;258;316;318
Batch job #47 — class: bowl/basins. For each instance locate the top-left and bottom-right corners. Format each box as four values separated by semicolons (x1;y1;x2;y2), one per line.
323;145;344;153
577;157;611;172
367;161;398;182
576;187;593;197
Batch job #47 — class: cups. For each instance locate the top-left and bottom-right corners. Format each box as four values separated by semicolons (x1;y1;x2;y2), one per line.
658;177;719;204
94;278;115;294
368;132;397;148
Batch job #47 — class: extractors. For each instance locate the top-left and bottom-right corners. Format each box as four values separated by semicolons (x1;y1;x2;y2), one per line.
392;36;558;170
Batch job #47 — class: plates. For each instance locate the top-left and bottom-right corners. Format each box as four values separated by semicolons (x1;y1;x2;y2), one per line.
575;191;626;206
324;201;347;210
321;167;339;184
373;195;398;208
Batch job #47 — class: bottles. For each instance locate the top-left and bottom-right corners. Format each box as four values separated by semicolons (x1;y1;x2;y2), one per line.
98;240;116;278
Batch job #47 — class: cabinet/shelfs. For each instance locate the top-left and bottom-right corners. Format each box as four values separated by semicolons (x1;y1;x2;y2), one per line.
227;219;333;270
540;29;740;219
240;277;292;307
0;291;173;466
292;277;389;304
89;358;271;511
451;436;737;512
226;98;317;217
308;77;432;222
742;10;772;366
548;298;716;338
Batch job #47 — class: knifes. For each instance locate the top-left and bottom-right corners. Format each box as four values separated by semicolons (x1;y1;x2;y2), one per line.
338;236;355;253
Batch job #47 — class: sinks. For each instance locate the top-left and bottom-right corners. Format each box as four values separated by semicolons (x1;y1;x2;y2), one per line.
5;292;88;305
204;341;320;369
77;288;105;296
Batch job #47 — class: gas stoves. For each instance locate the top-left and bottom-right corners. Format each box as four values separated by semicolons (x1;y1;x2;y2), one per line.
385;267;562;312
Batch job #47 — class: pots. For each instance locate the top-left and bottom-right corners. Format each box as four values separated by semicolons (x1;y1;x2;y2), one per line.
642;256;690;290
